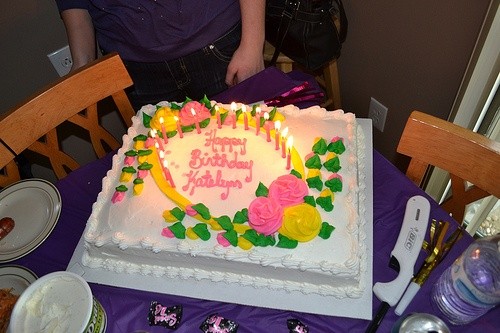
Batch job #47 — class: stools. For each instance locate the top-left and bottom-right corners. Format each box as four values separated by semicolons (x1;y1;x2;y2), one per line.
261;16;344;112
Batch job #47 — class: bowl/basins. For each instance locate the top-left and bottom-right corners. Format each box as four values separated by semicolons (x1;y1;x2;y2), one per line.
8;270;107;333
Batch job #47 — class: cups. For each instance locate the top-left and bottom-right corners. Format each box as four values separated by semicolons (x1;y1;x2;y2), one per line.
431;233;500;327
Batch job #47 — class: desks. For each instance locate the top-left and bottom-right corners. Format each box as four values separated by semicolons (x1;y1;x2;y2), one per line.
0;53;138;188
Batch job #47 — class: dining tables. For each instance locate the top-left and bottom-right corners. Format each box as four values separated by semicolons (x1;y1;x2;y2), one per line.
0;121;500;333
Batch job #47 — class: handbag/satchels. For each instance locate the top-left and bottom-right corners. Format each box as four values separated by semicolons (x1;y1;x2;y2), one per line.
263;0;348;71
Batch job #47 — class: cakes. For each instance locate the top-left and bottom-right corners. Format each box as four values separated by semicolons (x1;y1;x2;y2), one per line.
81;94;368;299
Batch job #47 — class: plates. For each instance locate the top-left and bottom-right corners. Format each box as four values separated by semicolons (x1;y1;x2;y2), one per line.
0;179;61;266
1;266;39;296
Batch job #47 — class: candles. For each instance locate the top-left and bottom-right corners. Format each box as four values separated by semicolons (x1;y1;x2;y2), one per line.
149;102;295;188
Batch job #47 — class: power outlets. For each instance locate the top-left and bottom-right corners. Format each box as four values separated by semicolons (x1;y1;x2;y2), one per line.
368;97;389;132
47;46;73;76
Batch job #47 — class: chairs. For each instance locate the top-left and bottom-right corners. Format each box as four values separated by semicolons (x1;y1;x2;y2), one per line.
397;111;500;225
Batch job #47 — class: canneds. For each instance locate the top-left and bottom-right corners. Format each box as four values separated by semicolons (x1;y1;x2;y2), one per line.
393;313;452;333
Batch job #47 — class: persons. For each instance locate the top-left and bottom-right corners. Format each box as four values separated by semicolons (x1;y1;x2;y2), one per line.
54;0;266;117
